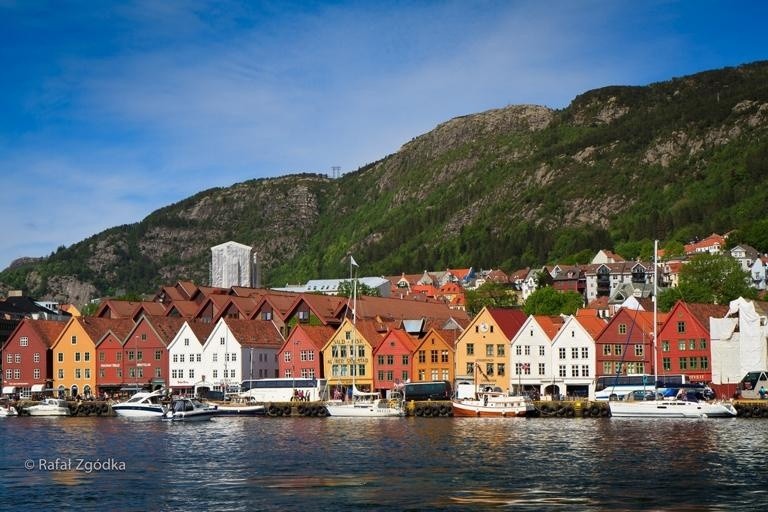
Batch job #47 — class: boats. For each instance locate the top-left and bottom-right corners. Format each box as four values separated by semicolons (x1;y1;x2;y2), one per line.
0;396;19;418
161;394;216;423
21;397;71;418
110;387;166;420
208;403;267;416
450;390;535;419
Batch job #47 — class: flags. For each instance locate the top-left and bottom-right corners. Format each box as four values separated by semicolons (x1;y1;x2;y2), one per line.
350;255;360;268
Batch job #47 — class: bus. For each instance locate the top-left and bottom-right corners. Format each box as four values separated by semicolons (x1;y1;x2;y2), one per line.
237;377;328;404
594;373;685;401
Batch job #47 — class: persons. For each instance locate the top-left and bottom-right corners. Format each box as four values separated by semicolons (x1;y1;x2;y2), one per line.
12;392;133;403
757;386;765;399
294;389;311;401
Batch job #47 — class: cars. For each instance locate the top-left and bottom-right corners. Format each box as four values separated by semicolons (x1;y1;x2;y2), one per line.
682;381;714;400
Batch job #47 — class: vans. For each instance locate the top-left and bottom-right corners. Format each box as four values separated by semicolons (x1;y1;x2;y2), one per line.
395;380;451;401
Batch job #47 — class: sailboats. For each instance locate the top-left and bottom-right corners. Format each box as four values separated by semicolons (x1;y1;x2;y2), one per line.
320;255;408;419
606;239;739;419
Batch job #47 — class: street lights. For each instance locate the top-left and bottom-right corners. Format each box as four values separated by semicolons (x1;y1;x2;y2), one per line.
248;348;256;406
134;335;140;393
